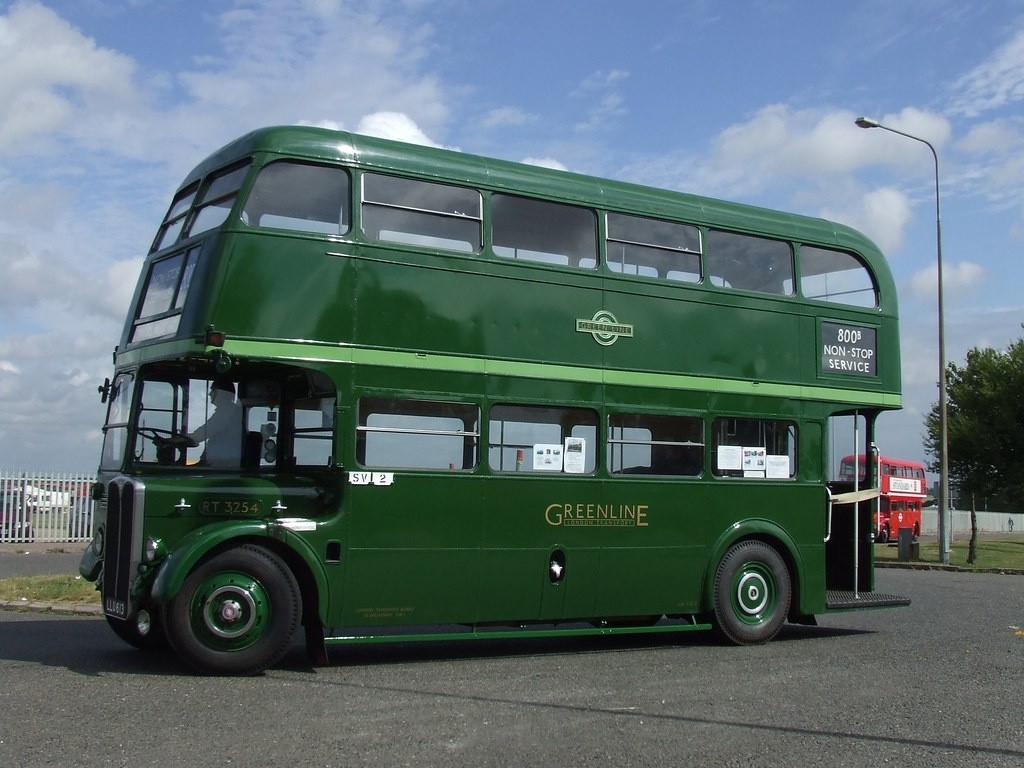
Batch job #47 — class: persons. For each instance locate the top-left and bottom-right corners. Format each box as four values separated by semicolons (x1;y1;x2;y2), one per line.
173;380;242;467
1008;517;1014;531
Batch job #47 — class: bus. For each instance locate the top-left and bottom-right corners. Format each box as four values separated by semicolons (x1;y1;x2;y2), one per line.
838;454;930;543
78;124;906;680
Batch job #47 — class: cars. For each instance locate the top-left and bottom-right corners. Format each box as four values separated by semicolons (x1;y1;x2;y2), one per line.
1;483;93;543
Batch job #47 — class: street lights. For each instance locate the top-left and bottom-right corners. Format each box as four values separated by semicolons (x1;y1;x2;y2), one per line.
855;117;953;567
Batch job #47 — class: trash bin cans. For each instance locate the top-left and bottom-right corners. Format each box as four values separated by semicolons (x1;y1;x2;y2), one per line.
897;526;912;560
